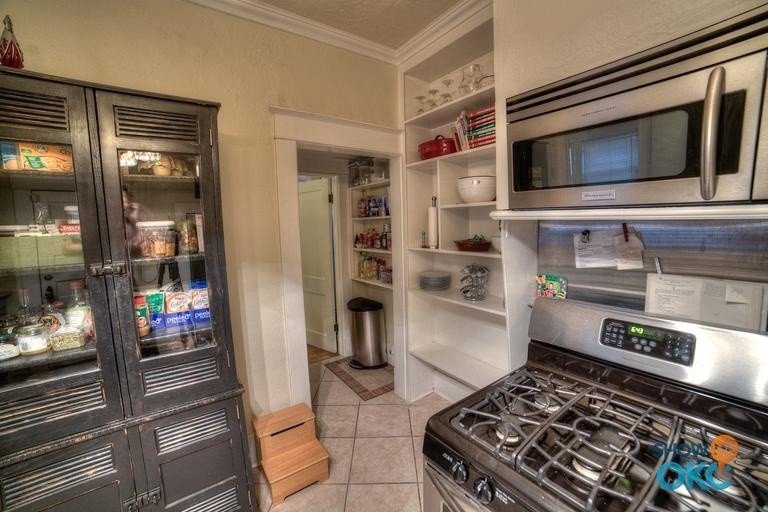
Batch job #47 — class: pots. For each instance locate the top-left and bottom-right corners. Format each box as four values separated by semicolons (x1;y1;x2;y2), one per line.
418;135;458;159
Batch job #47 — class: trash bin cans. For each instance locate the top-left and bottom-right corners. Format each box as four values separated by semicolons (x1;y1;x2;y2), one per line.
346;296;389;370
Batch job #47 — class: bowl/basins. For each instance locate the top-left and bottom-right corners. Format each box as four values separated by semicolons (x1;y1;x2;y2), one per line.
477;74;495;89
453;239;492;252
456;175;496;203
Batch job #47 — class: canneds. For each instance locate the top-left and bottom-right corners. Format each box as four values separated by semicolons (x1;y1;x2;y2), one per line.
0;333;21;361
0;313;21;347
18;322;51;356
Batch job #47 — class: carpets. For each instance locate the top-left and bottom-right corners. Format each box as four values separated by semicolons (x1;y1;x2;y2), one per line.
324;353;394;402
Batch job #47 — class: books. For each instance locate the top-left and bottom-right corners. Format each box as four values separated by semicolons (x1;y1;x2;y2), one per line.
463;107;496;146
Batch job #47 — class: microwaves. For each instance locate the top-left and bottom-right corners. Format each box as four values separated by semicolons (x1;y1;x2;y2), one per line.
503;3;768;211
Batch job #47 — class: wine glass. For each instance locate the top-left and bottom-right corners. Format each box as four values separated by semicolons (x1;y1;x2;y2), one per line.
414;76;454;116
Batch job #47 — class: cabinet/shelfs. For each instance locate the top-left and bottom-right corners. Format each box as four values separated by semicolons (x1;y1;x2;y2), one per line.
346;179;393;290
0;68;261;511
404;17;511;408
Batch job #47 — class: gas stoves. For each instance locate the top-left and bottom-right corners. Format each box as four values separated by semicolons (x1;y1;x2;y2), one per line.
423;361;767;511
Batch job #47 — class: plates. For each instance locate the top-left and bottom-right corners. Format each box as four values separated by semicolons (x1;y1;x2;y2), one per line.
419;270;451;292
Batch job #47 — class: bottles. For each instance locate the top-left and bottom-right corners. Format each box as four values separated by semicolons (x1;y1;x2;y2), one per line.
355;191;391;282
69;281;85;309
13;288;41;327
135;220;198;258
420;196;437;250
458;64;484;97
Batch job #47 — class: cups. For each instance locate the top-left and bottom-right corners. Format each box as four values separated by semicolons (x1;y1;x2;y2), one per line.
152;154;188;178
64;205;80;224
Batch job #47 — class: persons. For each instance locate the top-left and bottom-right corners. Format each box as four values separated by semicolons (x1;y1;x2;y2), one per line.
546;282;556;296
